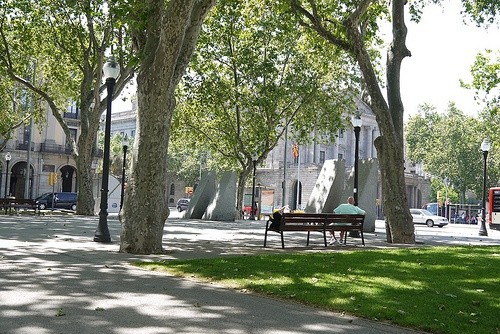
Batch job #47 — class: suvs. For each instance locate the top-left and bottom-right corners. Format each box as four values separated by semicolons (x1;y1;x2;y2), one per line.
176;198;191;212
32;192;79;211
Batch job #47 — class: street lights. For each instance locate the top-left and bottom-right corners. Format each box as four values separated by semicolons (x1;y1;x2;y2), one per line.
249;150;260;220
119;133;130;222
479;137;490;236
348;106;363;238
93;50;121;243
5;150;12;215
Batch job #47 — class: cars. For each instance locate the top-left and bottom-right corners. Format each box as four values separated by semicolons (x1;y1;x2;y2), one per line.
409;209;448;227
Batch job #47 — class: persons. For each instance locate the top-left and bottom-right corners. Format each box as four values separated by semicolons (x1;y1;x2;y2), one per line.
328;196;367;246
460;212;481;224
53;193;60;208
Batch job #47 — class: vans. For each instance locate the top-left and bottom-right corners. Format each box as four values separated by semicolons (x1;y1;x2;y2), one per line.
243;194;258;215
422;203;440;214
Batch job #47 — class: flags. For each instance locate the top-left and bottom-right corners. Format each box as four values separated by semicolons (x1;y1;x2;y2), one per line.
292;142;300;159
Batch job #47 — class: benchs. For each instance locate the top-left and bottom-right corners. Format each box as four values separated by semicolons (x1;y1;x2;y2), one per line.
263;209;366;250
0;197;39;216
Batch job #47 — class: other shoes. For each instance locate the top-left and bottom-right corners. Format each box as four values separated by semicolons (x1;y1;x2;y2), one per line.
339;239;344;245
330;238;337;245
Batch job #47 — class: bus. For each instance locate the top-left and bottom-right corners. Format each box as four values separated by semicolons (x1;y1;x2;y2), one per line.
487;187;500;230
447;203;480;224
